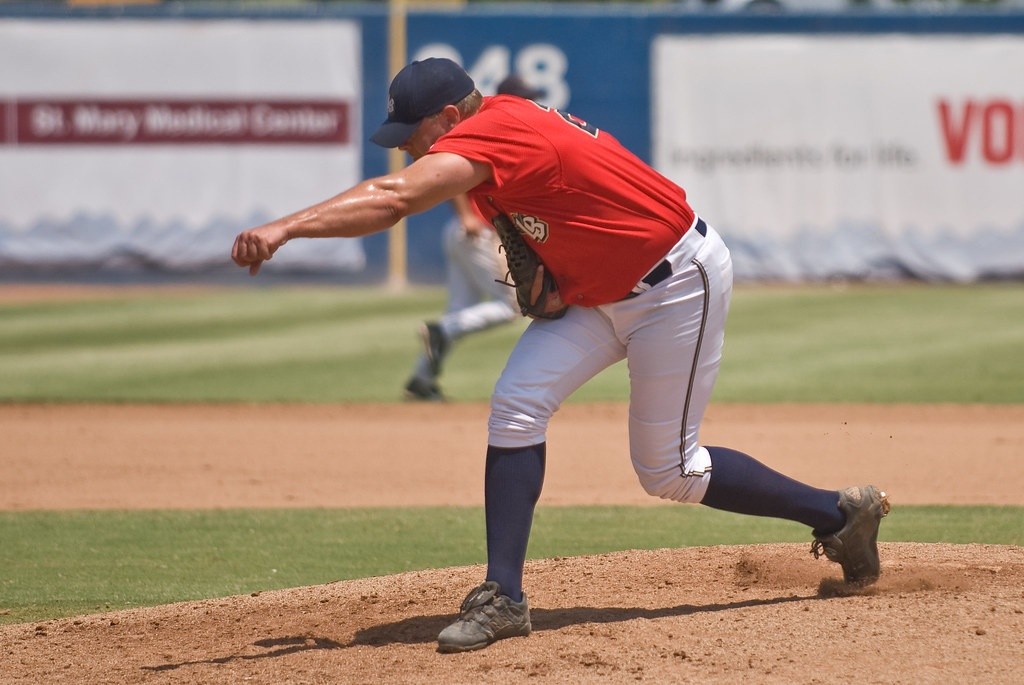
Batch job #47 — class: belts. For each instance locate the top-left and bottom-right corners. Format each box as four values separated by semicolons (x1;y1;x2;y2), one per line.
624;216;707;300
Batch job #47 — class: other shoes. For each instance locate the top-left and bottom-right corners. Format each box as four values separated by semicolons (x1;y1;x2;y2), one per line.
406;377;456;403
421;322;444;375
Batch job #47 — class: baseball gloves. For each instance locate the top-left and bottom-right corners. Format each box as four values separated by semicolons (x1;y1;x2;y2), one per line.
492;213;569;321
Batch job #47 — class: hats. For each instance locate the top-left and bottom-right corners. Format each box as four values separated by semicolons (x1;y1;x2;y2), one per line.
369;57;474;148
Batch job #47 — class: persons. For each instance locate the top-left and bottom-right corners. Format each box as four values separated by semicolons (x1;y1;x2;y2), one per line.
406;75;548;402
231;57;890;652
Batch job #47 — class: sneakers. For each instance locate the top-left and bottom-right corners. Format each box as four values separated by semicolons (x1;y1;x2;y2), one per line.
438;581;531;652
810;485;890;588
